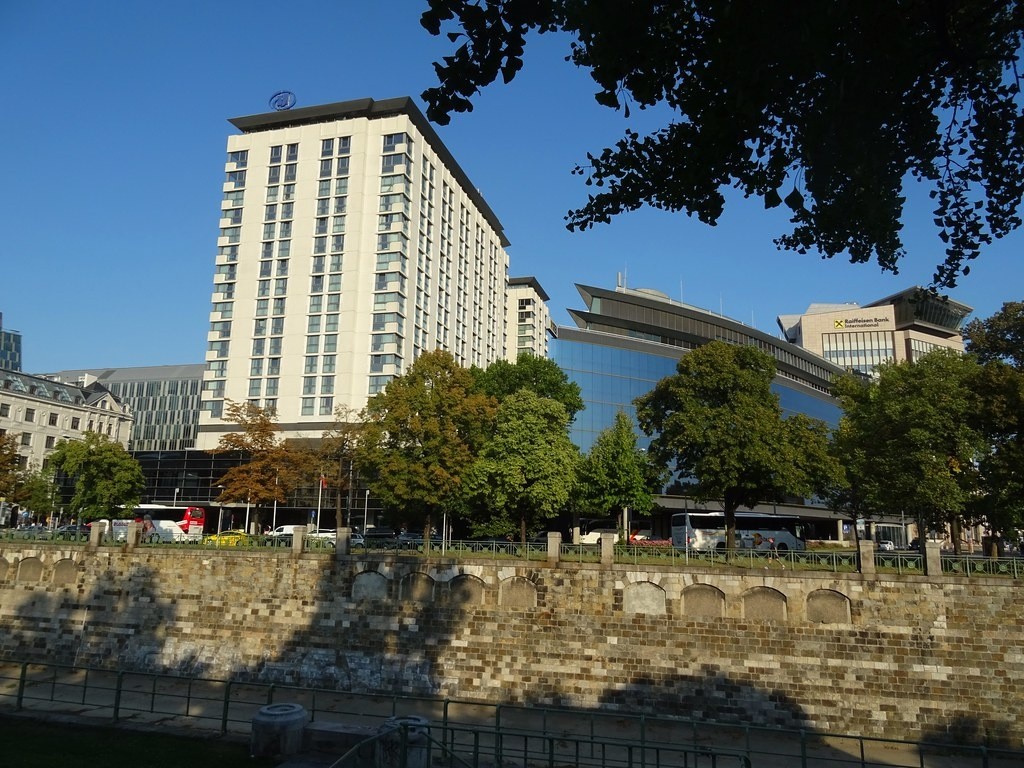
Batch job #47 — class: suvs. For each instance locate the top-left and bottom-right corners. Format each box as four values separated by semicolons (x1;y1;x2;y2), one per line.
306;528;338;548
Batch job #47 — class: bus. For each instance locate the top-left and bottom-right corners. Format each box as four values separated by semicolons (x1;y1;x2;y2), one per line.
671;513;807;555
78;504;206;544
626;519;654;542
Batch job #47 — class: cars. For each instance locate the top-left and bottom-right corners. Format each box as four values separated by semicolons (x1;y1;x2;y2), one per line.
394;533;446;550
580;528;621;545
0;522;92;541
202;530;254;546
350;533;365;549
878;539;894;552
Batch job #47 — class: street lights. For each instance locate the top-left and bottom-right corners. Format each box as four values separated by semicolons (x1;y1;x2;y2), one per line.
214;484;224;548
362;489;371;537
174;487;180;507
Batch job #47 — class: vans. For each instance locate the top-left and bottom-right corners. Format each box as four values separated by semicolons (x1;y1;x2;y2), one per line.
268;524;305;537
